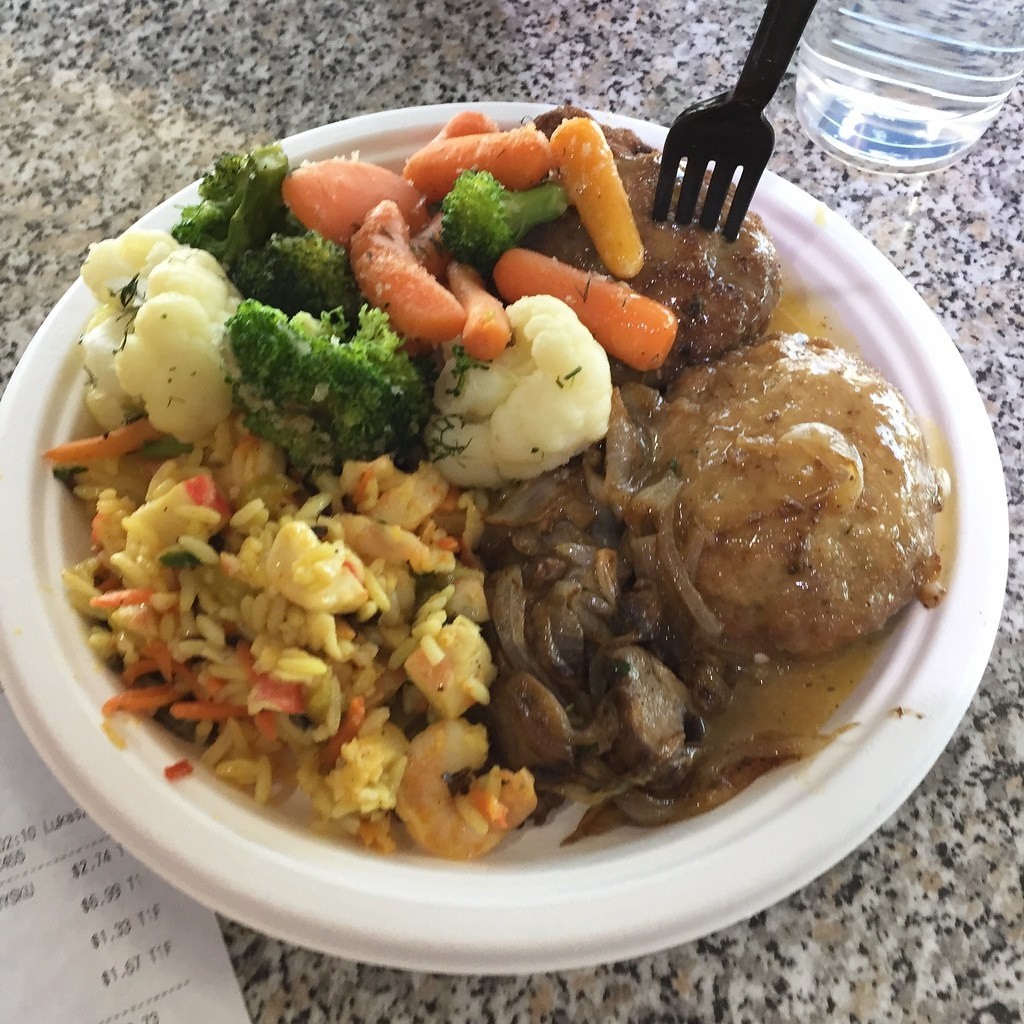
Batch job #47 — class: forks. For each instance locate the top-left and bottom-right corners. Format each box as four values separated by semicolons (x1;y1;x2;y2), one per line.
651;0;818;244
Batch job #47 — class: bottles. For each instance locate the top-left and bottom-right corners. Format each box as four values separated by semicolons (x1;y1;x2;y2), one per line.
795;1;1024;180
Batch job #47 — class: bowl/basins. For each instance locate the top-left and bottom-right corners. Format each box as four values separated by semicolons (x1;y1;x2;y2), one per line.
1;100;1010;978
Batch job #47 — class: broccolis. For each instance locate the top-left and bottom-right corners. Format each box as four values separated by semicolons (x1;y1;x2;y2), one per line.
79;145;616;486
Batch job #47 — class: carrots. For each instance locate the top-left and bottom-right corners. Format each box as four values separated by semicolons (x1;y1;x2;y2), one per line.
281;109;676;376
48;420;465;764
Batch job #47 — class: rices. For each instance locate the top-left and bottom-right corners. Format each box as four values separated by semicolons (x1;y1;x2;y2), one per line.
73;418;511;850
